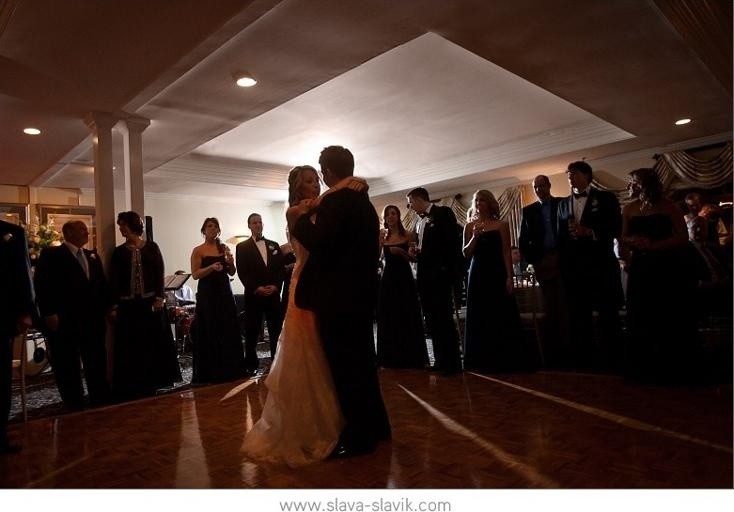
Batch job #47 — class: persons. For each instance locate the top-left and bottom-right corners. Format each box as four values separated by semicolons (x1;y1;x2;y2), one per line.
617;168;690;384
406;186;463;372
0;210;184;460
190;217;247;386
235;212;284;375
557;161;626;366
292;144;393;463
461;188;522;371
238;163;370;466
279;222;297;319
510;247;532;274
517;175;593;369
683;194;733;289
376;204;430;369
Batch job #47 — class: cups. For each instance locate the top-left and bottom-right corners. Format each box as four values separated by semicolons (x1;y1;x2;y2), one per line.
410;242;418;256
223;250;232;268
567;217;577;232
472;220;483;233
380;228;388;244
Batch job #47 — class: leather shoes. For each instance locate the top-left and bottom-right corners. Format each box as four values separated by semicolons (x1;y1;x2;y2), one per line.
331;442;353;458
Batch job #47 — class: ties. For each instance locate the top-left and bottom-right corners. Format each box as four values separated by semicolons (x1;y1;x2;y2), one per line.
76;249;87;274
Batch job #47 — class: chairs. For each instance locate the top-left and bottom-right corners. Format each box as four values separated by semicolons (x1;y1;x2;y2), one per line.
12;328;28;421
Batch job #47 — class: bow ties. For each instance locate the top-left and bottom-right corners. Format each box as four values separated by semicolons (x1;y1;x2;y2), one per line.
420;212;428;219
256;236;265;242
573;190;589;199
